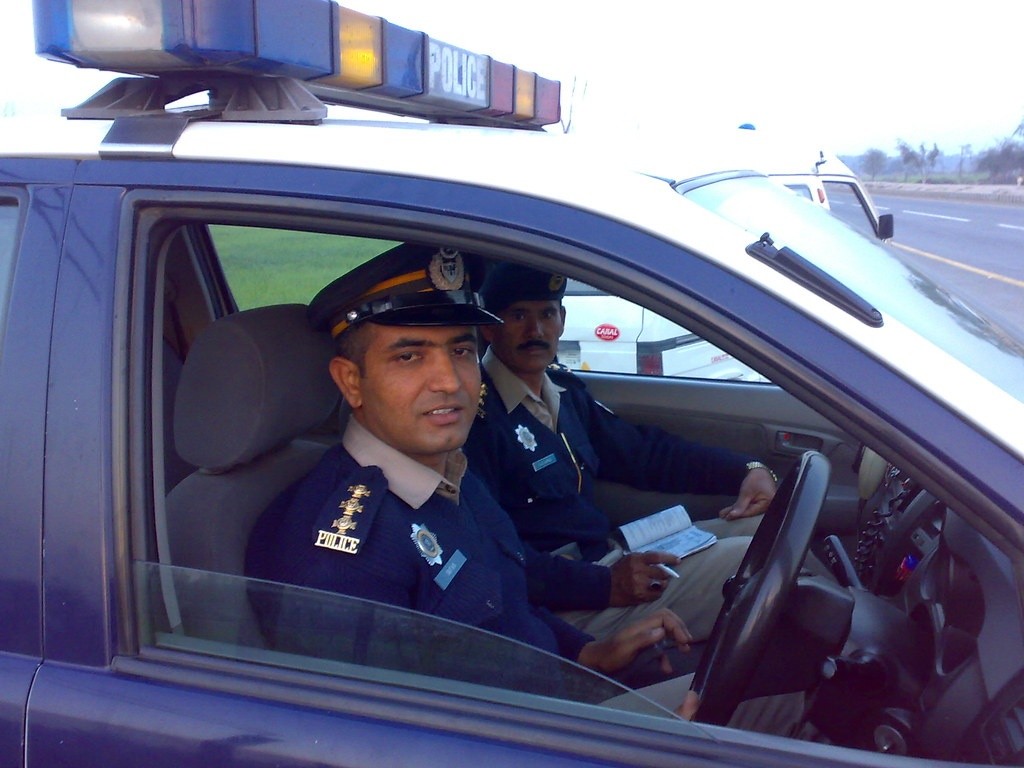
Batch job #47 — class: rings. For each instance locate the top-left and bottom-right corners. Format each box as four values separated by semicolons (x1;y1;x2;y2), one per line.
647;578;662;593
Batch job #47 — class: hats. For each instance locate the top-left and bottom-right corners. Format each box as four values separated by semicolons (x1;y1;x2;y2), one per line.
478;260;567;315
307;243;504;343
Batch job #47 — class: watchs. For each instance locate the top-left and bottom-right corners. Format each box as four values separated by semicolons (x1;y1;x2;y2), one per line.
744;462;779;489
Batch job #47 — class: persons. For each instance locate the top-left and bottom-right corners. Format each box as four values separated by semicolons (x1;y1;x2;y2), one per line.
243;241;838;748
461;259;812;650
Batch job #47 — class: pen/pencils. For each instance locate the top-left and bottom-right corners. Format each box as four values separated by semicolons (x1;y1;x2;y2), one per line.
622;551;680;580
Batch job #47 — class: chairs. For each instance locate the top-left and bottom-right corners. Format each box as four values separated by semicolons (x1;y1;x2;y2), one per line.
165;303;341;645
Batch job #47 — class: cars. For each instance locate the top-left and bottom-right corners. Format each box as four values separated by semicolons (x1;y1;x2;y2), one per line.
1;1;1024;767
557;273;765;385
733;121;894;246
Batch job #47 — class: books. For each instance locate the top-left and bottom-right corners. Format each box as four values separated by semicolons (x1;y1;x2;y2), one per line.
617;504;717;564
